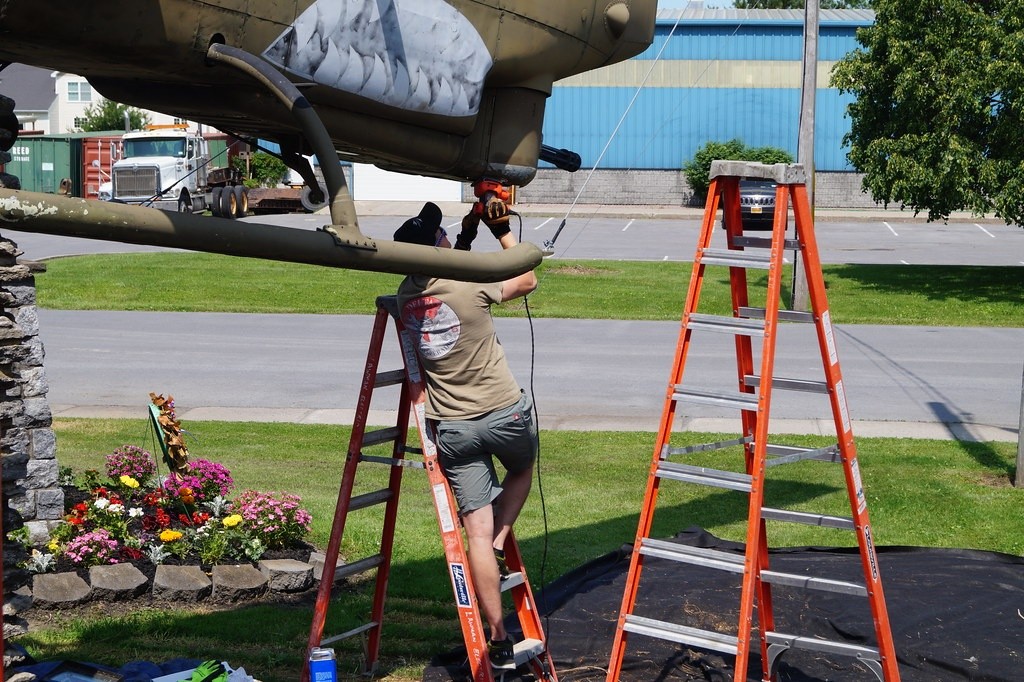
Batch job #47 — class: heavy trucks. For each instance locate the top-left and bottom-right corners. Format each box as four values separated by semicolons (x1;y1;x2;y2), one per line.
92;110;249;219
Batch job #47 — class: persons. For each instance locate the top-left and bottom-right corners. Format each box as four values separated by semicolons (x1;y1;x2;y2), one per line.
392;193;538;670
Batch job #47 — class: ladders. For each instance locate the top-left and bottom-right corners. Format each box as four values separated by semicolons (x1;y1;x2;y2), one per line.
301;293;565;682
604;157;910;682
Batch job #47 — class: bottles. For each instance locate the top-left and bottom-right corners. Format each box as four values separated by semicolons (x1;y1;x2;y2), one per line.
309;646;338;682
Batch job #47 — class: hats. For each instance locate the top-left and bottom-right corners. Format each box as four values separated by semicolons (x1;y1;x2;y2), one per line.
394;202;442;246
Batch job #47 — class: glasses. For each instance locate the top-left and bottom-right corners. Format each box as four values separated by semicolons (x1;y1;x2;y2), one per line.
435;227;447;246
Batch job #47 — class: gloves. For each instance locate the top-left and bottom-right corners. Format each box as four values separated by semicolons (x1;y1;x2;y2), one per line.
461;202;481;242
482;197;511;239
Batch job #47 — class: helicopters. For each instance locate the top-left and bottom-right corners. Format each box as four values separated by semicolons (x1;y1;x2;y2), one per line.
1;0;659;283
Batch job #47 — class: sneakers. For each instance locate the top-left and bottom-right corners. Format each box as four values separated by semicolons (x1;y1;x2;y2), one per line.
493;547;510;579
487;639;516;670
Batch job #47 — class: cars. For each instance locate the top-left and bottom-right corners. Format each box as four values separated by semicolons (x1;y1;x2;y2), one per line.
721;180;788;230
